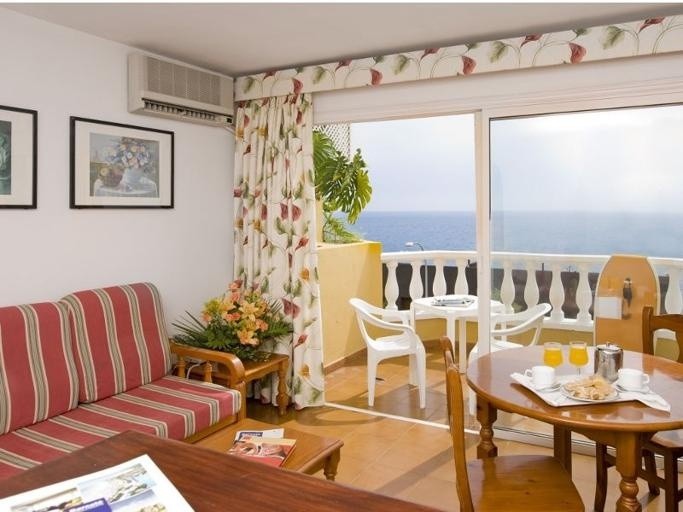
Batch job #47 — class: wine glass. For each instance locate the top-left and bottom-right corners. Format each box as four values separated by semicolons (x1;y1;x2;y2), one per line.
568;339;590;382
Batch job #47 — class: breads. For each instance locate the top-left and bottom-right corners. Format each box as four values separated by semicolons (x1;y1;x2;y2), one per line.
569;378;611;402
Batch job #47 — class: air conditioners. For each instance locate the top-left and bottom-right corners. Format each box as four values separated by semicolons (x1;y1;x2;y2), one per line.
126;51;235;132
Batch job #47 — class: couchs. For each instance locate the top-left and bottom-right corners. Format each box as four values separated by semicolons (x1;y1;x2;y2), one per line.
0;279;249;478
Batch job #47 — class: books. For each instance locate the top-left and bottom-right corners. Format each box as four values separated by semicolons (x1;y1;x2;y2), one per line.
226;427;296;468
430;297;475;307
0;454;195;512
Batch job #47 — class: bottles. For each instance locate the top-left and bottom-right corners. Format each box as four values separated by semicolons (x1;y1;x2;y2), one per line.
593;341;624;381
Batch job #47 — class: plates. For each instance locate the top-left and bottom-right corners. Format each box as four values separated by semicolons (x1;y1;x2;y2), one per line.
611;382;652;394
561;382;620;401
542;381;562;393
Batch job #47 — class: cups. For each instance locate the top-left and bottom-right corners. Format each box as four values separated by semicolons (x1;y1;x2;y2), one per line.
524;365;556;389
617;366;651;390
543;342;563;368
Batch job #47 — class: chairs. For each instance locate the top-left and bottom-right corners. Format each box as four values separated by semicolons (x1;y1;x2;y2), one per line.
593;304;681;512
466;302;551;420
346;294;429;412
434;332;586;512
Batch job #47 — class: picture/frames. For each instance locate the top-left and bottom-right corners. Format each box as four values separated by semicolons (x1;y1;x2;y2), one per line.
66;114;176;211
0;103;42;211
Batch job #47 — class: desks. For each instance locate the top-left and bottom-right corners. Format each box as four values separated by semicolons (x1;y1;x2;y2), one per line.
1;427;448;512
407;294;510;399
182;350;290;420
462;340;680;512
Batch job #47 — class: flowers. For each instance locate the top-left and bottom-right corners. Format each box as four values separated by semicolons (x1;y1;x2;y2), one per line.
171;277;295;365
